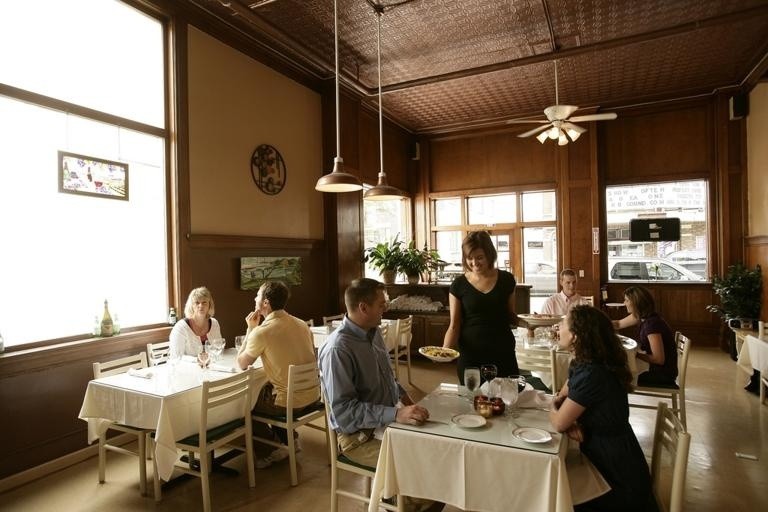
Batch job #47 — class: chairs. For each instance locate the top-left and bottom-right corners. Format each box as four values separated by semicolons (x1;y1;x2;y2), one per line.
516;349;557;394
630;331;691;432
174;365;255;512
391;314;412;384
92;351;155;496
323;314;344;325
147;340;169;367
321;377;403;512
651;401;691;511
305;319;315;326
380;325;393;359
251;361;331;487
758;317;768;405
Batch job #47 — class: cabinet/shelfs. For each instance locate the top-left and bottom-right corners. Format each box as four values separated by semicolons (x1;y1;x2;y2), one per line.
382;284;452;362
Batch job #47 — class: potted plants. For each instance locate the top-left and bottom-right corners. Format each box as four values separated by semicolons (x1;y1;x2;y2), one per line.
705;261;763;361
362;229;406;284
397;237;447;285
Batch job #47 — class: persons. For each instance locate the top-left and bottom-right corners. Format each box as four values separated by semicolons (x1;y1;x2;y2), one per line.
541;268;592;318
237;281;321;469
610;285;679;385
316;278;446;511
430;231;552;388
548;305;661;511
166;286;222;364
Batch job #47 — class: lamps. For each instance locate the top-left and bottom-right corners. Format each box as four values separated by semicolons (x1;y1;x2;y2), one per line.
503;58;619;147
360;0;410;203
536;122;583;147
312;1;366;197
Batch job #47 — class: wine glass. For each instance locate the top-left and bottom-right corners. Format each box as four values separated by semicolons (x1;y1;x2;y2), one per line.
533;328;562;346
464;367;480;406
481;365;528;422
193;336;245;371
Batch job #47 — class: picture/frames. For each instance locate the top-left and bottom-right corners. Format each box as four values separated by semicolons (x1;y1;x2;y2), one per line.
56;148;130;202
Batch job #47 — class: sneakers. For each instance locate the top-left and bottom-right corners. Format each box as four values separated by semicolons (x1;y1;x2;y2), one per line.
255;437;301;470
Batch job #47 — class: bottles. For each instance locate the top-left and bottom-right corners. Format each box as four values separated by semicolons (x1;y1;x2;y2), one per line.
101;299;113;337
93;314;102;336
0;332;6;356
169;307;176;326
112;313;120;335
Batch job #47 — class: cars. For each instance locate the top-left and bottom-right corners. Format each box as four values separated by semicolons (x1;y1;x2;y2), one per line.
434;225;709;282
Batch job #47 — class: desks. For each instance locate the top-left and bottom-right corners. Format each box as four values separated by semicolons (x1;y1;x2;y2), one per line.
737;334;768;404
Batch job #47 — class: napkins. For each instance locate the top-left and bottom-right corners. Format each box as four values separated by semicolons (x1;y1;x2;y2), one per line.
309;327;328;332
482;378;552;408
128;366;154;379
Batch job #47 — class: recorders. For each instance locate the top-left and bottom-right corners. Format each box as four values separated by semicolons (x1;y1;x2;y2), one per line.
728;317;758;330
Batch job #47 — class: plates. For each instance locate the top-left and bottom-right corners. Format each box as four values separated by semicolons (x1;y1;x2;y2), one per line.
449;412;486;429
512;426;553;445
418;345;460;362
517;313;562;326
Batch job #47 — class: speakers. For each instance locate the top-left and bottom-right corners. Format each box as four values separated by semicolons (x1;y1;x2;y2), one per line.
728;95;745;121
410;142;420;161
